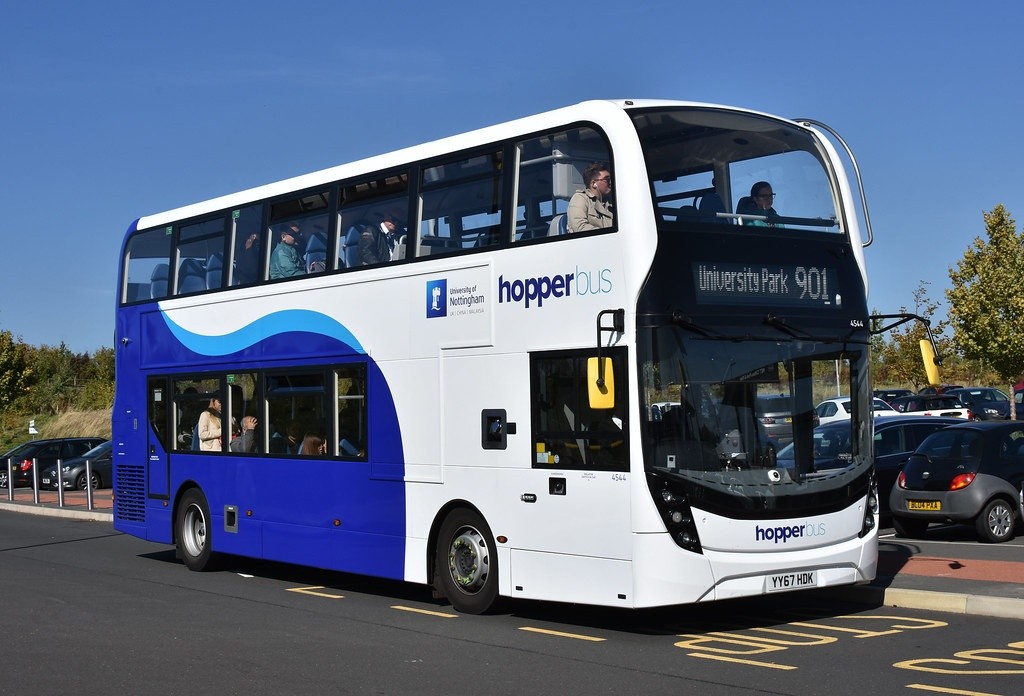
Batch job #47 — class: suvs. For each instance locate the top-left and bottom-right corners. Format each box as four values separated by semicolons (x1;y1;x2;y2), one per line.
0;436;109;489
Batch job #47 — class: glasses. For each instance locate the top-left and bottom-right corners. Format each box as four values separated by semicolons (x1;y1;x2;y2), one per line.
388;215;402;225
754;193;776;201
592;178;611;185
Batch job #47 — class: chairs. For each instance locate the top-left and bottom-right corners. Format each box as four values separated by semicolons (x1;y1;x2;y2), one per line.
298;440;303;455
474;224;501;247
149;263;170;299
206;252;222;289
191;423;200;451
272;432;292;455
547;214;567;238
736;197;778;216
968;438;983;459
232;385;244;416
177;258;207;295
676;206;698;221
306;232;328;273
1007;436;1024;457
344;224;365;267
182;387;200;430
698;193;730;225
340;439;360;456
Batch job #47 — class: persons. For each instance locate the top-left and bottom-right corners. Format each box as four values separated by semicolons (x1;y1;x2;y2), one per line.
339;385;366;458
278;420;328;455
567;161;613;233
231;415;258;453
269;222;317;279
737;181;785;228
661;387;707;441
198;390;238;452
360;207;403;266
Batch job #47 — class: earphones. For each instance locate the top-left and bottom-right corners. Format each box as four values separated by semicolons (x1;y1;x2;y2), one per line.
594;183;597;186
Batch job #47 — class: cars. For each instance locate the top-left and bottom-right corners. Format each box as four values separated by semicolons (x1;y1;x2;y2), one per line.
33;440;112;491
647;383;1024;544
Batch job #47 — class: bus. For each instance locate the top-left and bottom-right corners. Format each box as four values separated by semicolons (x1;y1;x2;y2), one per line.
111;99;945;615
